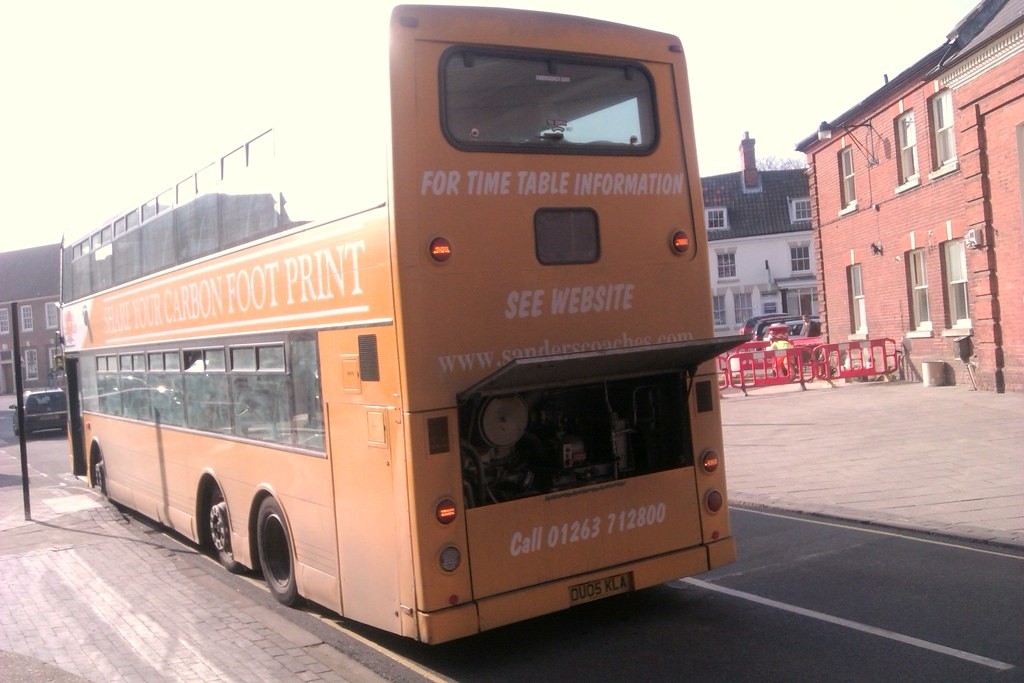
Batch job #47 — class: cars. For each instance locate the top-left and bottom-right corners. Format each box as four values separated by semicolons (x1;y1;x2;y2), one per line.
738;313;821;342
78;375;184;420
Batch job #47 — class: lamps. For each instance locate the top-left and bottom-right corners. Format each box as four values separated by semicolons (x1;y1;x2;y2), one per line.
817;120;879;167
871;242;882;256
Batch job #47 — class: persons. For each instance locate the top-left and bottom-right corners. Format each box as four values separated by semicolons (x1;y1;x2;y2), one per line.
799;314;819;337
48;369;57;389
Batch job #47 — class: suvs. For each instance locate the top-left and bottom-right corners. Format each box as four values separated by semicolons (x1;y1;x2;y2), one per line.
7;386;69;438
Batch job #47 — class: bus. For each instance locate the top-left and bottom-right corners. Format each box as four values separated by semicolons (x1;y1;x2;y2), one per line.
48;0;758;647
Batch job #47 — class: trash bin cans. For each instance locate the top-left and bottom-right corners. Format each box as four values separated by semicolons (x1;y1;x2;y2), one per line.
922;361;946;387
